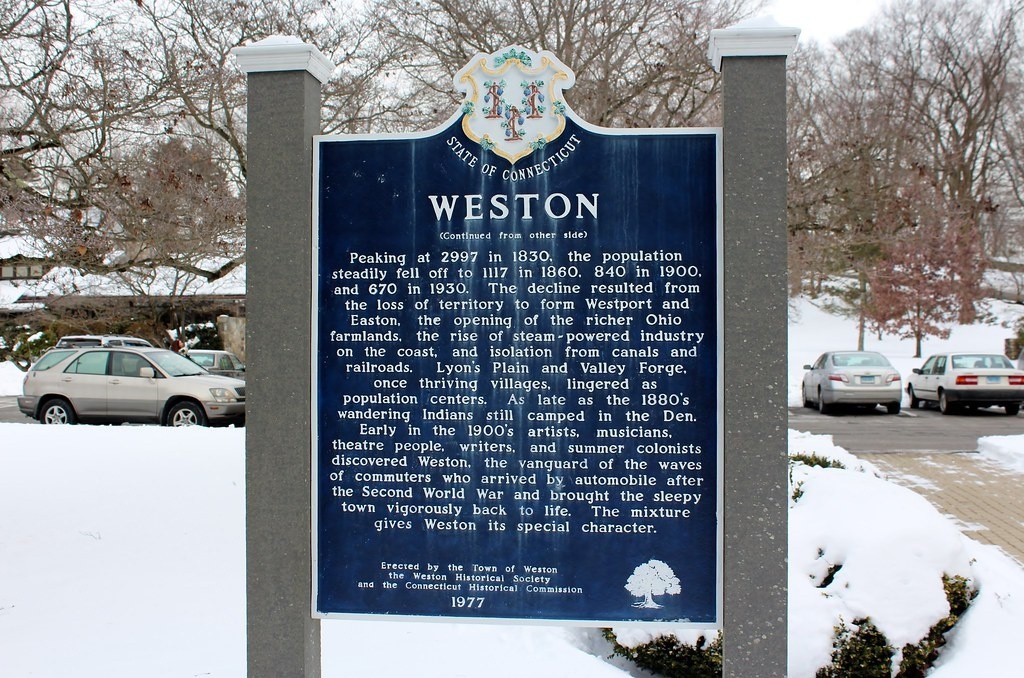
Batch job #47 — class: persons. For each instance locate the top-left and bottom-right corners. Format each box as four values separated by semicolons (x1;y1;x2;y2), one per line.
171;339;188;357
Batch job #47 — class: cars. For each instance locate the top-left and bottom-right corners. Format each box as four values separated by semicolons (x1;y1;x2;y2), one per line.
17;344;245;427
187;350;245;381
905;352;1024;416
801;351;902;414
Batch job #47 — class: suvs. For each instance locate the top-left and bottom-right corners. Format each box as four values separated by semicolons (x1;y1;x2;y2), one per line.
56;334;153;372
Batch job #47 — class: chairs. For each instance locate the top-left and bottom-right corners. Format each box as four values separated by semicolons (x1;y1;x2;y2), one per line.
135;358;151;375
975;361;985;368
